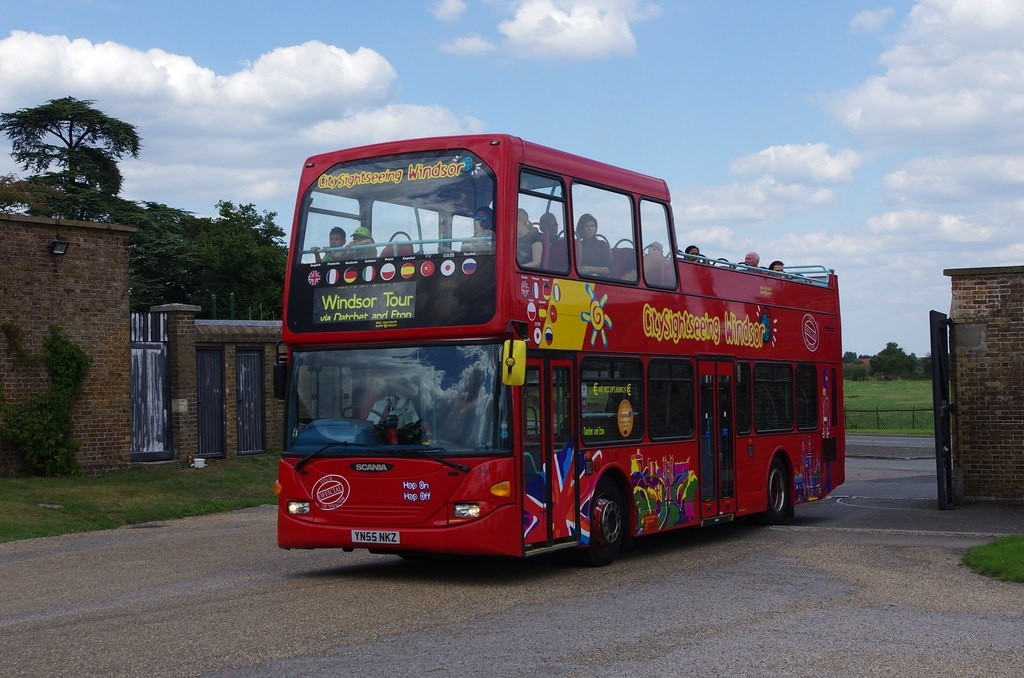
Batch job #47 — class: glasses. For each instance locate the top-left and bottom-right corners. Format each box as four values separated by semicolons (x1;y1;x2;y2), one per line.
474;216;488;222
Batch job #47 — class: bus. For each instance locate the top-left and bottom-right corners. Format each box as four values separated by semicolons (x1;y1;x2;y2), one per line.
271;134;844;568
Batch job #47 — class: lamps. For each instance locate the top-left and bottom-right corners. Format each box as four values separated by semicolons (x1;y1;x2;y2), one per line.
48;239;70;254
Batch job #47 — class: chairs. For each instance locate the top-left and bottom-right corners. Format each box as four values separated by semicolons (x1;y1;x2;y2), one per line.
529;223;807;286
738;378;810;428
380;231;415;257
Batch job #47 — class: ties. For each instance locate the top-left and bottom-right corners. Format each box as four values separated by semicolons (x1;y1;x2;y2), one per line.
379;398;393;425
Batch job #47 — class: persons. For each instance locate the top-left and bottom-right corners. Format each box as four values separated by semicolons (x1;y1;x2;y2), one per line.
310;206;785;287
359;370;419;444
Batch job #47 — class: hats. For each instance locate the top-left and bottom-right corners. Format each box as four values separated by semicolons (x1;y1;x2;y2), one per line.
350;226;371;238
